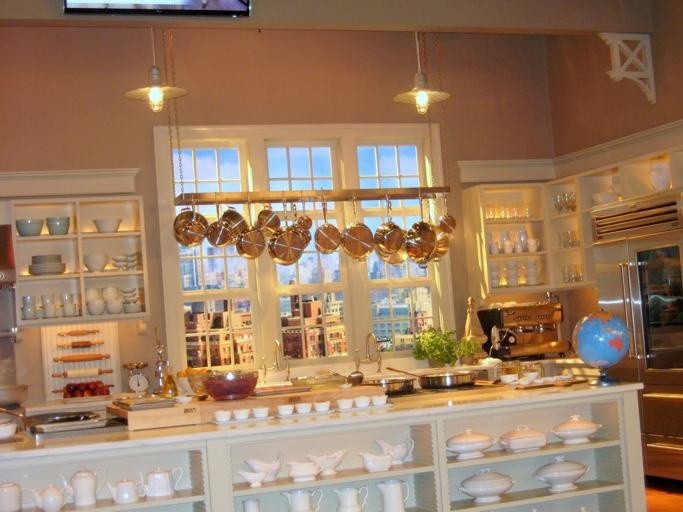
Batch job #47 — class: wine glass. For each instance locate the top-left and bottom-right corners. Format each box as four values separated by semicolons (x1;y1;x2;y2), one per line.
121;293;137;298
112;255;127;262
112;262;128;271
128;261;138;272
122;297;140;303
128;256;137;262
553;192;576;214
120;287;137;293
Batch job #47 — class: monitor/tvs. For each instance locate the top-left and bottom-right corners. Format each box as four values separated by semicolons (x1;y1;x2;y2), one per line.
64;0;252;17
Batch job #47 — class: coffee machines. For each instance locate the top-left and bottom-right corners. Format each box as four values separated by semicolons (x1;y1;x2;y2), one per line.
476;304;570;359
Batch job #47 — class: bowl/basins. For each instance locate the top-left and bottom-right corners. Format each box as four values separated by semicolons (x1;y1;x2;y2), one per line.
85;287;99;302
93;219;122;232
200;370;259;401
59;292;77;317
16;219;44;236
22;296;37;320
491;258;539;287
84;254;105;272
186;371;209;396
87;300;105;315
106;300;122;313
101;287;119;300
41;294;58;319
123;304;142;313
47;217;70;234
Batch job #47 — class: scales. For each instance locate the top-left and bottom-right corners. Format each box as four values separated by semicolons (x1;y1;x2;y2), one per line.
123;361;149;400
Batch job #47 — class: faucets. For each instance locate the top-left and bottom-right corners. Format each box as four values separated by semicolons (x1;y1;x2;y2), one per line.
270;336;284;373
364;332;379;364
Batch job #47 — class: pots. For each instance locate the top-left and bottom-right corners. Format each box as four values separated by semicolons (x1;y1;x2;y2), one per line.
0;383;30;407
439;192;456;233
427;201;451;262
405;191;437;269
341;192;373;261
291;204;312;248
222;206;248;244
363;372;416;394
298;214;313;231
268;198;304;266
418;369;477;390
255;205;280;237
375;192;403;255
237;192;266;260
205;193;232;247
172;207;208;248
314;189;342;254
184;201;206;242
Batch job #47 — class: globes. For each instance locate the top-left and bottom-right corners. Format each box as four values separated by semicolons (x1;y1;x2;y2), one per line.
572;311;630;387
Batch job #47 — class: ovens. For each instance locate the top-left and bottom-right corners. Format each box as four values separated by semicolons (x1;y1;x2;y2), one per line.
591;190;682;453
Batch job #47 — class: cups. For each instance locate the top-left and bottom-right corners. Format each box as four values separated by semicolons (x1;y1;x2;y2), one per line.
559;264;584;283
252;406;269;418
313;400;330;411
235;471;265;487
502;372;571;388
233;408;249;419
295;403;312;413
355;396;369;407
214;411;231;421
306;448;348;476
372;395;387;405
486;203;530;218
276;404;294;414
337;399;352;408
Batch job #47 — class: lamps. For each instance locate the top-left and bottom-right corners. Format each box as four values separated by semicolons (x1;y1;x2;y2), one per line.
394;32;451;117
122;26;190;114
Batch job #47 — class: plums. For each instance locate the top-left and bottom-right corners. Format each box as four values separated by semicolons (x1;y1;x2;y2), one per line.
62;390;71;399
83;388;92;396
88;380;96;391
73;393;83;398
103;386;110;396
94;387;100;397
75;382;86;390
66;383;73;391
95;380;103;387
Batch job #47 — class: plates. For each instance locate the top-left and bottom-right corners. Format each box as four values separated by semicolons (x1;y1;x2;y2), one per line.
316;410;336;417
32;254;61;264
296;413;315;417
276;414;298;419
233;418;255;423
209;420;237;426
254;416;274;421
28;263;67;275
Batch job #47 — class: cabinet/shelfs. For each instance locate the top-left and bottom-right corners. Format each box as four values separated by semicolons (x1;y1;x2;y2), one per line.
637;386;683;482
591;229;683;386
9;190;151;328
494;280;600;359
460;182;554;308
551;137;678;290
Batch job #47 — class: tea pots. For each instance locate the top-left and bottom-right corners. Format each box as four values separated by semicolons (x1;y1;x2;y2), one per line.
136;464;184;497
240;454;281;482
527;238;539;252
242;498;261;512
32;484;70;512
373;438;414;465
107;478;147;505
61;463;106;507
357;450;392;473
280;488;324;512
556;229;579;249
374;479;410;512
282;458;325;482
0;479;33;512
332;485;369;512
